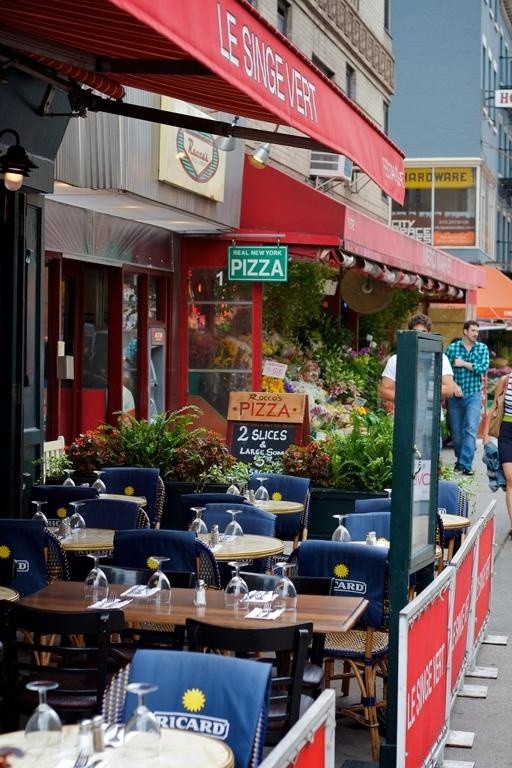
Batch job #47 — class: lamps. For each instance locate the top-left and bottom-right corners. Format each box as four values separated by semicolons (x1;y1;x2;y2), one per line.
212;113;242;151
250;123;286;170
0;130;39;192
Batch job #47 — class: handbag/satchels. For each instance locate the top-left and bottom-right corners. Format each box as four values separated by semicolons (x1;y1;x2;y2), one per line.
488;374;508;438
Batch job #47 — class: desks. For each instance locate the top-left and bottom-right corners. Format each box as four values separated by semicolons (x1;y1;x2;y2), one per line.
0;723;238;766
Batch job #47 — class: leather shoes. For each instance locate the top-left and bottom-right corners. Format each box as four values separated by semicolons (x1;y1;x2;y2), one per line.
454;465;475;475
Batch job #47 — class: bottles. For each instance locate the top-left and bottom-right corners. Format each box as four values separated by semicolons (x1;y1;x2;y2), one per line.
208;523;221;546
366;530;376;544
58;517;71;538
81;483;88;488
194;605;207;617
193;578;208;606
92;714;105;752
246;489;256;506
78;718;93;757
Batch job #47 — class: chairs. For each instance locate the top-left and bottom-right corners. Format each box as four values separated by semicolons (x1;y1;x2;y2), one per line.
1;459;466;748
100;646;275;767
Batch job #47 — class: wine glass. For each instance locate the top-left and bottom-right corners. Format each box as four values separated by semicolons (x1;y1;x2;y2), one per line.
24;678;64;755
69;500;86;534
147;555;171;603
90;470;108;497
222;559;253;609
224;507;246;547
187;505;207;535
254;476;275;502
82;551;111;602
382;486;392;498
331;512;350;541
63;468;75;488
271;561;301;611
227;475;241;496
30;499;48;524
123;681;163;760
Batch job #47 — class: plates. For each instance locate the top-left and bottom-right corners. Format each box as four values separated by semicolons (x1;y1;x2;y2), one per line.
53;723;137;768
87;596;135;610
243;498;268;508
198;532;243;553
52;525;82;544
120;583;160;598
240;588;286;621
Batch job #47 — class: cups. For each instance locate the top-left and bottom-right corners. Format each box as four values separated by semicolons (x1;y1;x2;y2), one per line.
147;603;171;617
437;506;447;523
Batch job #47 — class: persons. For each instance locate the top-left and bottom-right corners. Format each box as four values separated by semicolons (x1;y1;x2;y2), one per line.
442;319;491;476
121;385;136;420
481;370;512;539
377;312;456;454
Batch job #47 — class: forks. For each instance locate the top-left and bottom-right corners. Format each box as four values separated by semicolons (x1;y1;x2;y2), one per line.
99;591;115;609
106;596;133;608
